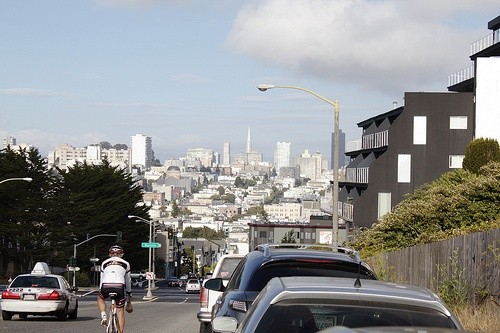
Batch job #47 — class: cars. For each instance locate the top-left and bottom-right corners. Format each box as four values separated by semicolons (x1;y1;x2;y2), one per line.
210;276;467;333
0;262;79;321
130;269;146;280
166;276;179;287
184;278;201;293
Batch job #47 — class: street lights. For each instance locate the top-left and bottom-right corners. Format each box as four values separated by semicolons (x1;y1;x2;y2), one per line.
257;84;340;254
136;220;155;291
128;215;153;298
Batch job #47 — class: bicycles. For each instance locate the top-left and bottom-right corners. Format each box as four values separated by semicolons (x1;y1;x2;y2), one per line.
101;292;126;333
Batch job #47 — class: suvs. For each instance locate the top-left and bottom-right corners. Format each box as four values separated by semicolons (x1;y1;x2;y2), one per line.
197;254;247;333
202;242;381;333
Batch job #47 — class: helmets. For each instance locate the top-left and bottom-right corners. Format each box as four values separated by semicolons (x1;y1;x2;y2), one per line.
109;245;124;257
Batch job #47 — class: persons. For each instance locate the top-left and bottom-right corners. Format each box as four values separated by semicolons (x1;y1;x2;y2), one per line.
98;245;132;333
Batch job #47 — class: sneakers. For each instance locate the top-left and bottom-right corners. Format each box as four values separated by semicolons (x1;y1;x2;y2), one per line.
100;315;107;325
